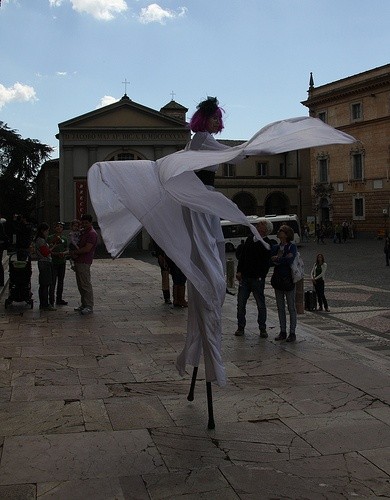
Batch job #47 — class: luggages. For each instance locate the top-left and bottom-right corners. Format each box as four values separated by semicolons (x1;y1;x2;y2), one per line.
304;279;318;311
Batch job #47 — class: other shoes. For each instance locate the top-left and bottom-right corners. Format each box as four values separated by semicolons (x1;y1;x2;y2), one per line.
285;334;297;342
164;299;172;304
56;299;68;305
274;332;288;340
74;305;93;315
318;306;323;311
325;308;330;312
235;329;244;336
39;304;52;309
260;330;269;338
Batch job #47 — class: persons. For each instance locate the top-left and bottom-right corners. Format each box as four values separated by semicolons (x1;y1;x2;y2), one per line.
15;216;98;314
151;238;188;309
311;253;331;312
384;231;390;266
235;219;273;337
300;219;354;244
182;97;272;310
270;225;298;342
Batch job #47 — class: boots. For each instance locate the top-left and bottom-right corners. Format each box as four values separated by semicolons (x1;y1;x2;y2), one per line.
173;285;188;307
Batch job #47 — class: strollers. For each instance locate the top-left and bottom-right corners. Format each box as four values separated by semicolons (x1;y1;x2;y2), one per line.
4;251;34;309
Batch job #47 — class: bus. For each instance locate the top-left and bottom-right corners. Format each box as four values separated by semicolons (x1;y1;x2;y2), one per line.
218;214;303;251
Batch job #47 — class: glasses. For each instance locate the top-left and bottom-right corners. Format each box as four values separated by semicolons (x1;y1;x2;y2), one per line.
279;228;285;233
